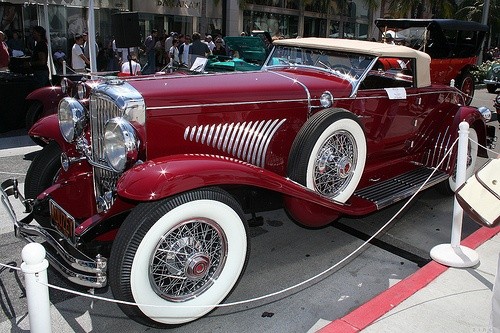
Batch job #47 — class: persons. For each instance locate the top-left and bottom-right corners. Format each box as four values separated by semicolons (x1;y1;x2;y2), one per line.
373;28;416;49
25;25;51;87
243;30;304;48
112;30;224;73
0;31;19;70
71;31;100;72
346;93;432;143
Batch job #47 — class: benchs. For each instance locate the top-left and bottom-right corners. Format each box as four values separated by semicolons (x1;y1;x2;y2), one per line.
352;70;413;92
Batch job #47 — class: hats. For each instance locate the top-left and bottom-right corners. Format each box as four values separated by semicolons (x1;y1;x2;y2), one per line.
206;35;212;39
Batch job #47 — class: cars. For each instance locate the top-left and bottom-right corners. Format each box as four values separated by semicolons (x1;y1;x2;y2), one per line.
0;36;496;329
203;35;289;72
357;15;490;107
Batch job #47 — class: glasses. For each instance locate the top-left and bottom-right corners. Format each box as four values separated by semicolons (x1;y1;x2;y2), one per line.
175;42;180;44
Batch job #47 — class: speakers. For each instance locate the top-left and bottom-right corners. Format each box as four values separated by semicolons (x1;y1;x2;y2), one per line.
111;11;142;48
